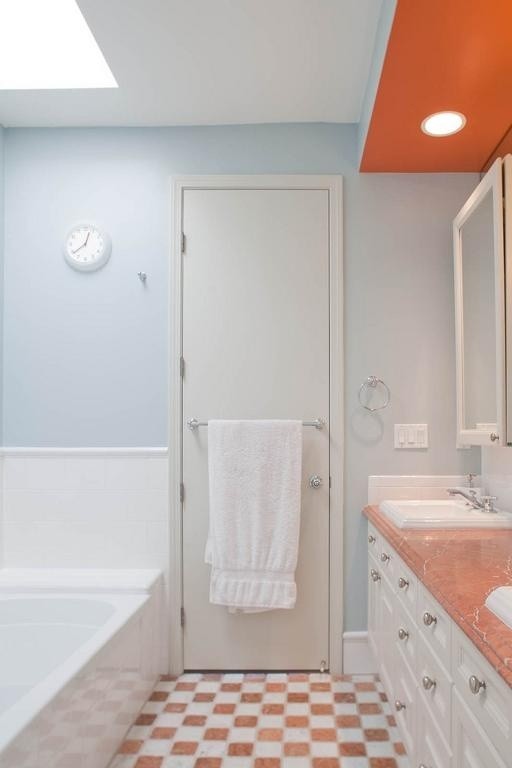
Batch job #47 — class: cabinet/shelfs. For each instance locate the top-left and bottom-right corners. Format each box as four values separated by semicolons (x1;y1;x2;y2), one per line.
367;520;512;768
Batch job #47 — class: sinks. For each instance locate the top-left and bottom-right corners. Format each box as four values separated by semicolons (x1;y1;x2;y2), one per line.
485;586;512;629
380;500;512;531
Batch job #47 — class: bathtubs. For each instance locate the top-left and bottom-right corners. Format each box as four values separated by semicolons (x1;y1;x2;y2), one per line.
0;594;156;768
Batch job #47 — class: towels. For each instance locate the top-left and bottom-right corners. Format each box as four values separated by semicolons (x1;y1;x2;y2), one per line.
207;420;303;614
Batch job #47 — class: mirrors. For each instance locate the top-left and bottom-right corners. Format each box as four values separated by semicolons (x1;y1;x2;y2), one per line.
451;154;511;448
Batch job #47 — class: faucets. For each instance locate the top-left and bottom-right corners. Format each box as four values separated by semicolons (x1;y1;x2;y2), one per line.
446;489;485;509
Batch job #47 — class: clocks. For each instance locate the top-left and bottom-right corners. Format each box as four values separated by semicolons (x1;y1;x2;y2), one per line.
64;225;111;273
